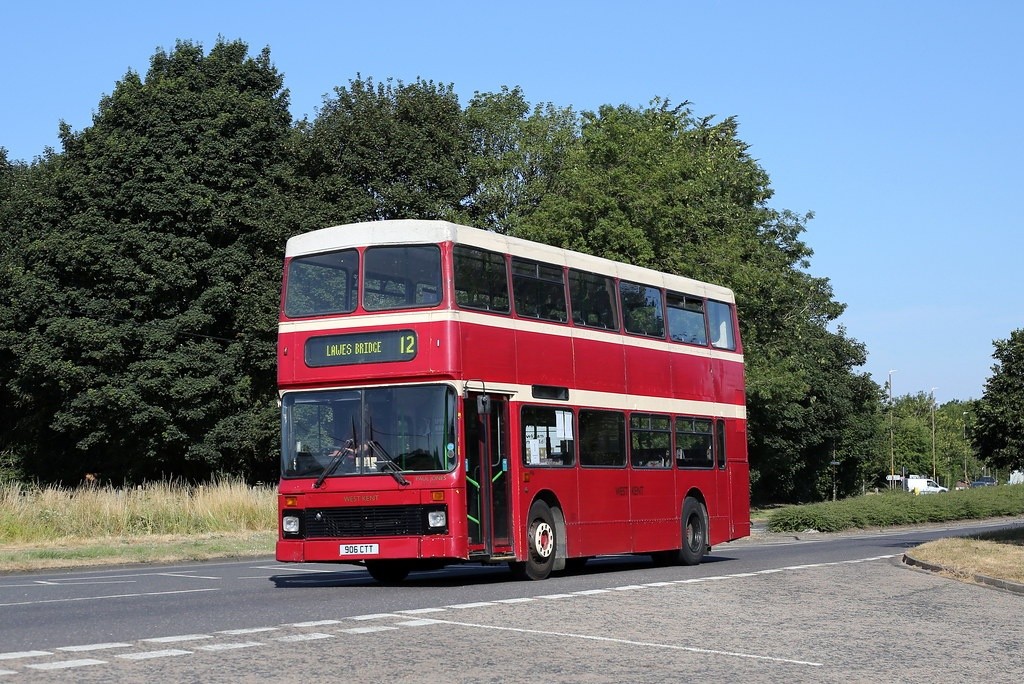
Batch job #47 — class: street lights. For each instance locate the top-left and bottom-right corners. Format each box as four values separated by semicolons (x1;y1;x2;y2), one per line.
888;369;900;490
963;411;969;482
931;387;941;482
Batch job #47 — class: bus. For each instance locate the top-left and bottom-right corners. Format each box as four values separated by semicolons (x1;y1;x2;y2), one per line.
275;219;751;580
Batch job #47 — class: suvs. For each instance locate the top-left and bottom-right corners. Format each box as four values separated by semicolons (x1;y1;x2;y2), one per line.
979;476;996;486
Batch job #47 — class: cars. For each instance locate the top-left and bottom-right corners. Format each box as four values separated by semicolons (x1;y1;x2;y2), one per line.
971;482;987;489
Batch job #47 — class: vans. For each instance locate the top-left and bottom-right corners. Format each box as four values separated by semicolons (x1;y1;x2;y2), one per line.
903;478;949;494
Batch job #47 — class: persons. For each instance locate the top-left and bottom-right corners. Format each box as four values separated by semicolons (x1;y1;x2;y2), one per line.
328;420;373;466
